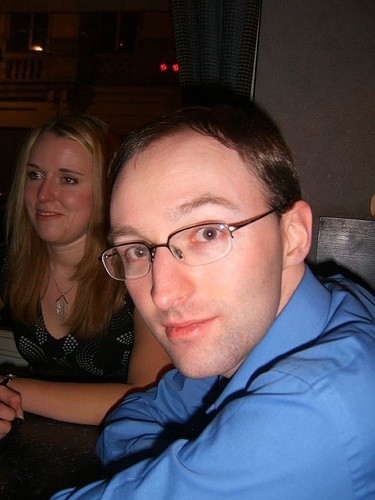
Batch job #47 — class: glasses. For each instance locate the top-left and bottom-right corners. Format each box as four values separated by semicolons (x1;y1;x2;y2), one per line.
95;200;281;281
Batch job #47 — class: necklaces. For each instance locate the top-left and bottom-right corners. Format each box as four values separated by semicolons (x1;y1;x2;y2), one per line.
52;266;84;322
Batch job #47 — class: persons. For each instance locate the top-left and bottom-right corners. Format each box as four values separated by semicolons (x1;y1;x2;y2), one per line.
0;103;178;453
27;78;374;500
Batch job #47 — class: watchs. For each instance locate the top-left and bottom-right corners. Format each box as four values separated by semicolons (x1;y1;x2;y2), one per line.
2;372;17;388
